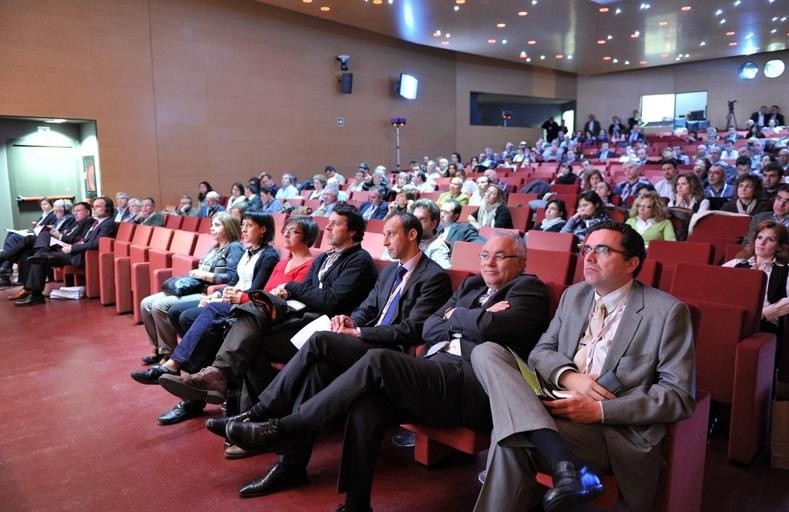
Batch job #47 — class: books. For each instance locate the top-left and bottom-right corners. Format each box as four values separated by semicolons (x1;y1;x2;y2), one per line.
535;368;558;400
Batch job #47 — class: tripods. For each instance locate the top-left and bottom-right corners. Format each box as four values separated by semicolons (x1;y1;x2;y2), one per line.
725;111;737;132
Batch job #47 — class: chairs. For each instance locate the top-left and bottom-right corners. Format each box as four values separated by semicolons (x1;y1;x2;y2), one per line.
54;126;789;512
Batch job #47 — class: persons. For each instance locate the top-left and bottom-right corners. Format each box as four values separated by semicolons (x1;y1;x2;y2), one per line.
519;159;709;251
681;107;787;166
130;197;164;226
158;205;378;460
205;212;451;498
297;162;514;244
470;221;697;512
121;198;142;222
225;170;299;214
226;232;549;512
2;197;117;304
702;155;787;305
197;181;213;210
423;109;646;169
131;216;318;425
172;193;197;216
197;192;225;216
168;213;279;374
114;192;130;221
709;221;789;449
142;212;244;368
659;143;690;165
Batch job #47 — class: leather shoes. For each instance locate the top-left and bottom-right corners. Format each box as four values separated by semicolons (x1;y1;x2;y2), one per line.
224;443;252;459
0;266;45;306
156;364;229;404
25;249;60;266
142;352;173;368
539;458;609;512
224;415;306;457
155;400;207;425
237;461;311;498
204;408;253;439
131;362;181;386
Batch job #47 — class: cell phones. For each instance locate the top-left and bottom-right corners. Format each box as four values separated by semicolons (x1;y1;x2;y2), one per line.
536;394;556;400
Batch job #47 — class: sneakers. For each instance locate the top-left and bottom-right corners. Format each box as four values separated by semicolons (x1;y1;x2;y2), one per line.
335;502;374;511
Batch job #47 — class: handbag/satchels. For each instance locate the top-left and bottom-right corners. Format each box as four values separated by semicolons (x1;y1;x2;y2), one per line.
159;272;205;298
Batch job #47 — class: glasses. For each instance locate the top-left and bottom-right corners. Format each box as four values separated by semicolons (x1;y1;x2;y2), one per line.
580;243;631;259
280;227;305;235
479;251;518;260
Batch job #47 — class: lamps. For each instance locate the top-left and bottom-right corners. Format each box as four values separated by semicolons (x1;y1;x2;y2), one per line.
336;55;353;70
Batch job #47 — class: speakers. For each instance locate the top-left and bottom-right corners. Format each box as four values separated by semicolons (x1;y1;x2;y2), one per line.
342;73;352;93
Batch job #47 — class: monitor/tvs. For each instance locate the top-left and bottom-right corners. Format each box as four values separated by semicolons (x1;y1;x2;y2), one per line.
399;73;418;99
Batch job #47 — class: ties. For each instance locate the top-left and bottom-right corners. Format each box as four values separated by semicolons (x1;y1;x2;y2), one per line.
423;289;498;359
563;297;609;375
378;265;409;328
76;219;99;247
